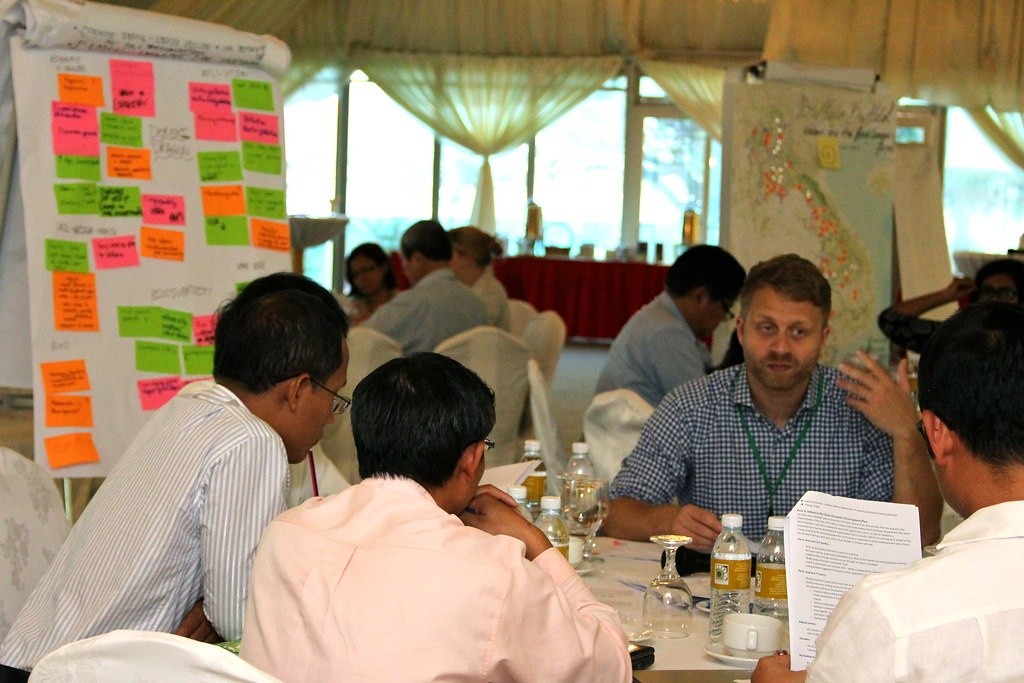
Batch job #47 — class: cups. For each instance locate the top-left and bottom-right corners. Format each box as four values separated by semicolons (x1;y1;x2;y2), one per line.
722;613;782;657
568;536;583;568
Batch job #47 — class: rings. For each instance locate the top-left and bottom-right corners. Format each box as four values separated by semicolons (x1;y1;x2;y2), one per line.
775;649;788;655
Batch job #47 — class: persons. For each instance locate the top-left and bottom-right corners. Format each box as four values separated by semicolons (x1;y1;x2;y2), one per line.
879;258;1024;353
345;243;400;325
582;253;942;548
352;219;485;355
750;301;1024;682
448;227;508;333
243;351;634;683
0;272;348;683
596;243;744;411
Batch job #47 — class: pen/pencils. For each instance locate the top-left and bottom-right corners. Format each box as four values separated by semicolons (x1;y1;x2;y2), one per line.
465;507;475;513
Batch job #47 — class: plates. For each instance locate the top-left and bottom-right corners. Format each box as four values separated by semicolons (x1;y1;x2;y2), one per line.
703;643;758;669
696;600;710;613
575;561;596;576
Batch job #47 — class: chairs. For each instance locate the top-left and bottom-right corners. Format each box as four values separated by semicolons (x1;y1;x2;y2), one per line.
525;358;569;498
433;326;530;465
26;628;285;683
509;298;539;340
0;442;71;641
583;387;679;506
319;326;407;464
522;310;565;431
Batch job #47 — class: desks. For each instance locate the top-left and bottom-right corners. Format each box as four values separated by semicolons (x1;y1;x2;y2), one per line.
499;255;668;339
554;530;782;683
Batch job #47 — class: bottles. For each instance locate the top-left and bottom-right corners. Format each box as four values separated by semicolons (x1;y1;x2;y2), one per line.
754;516;789;641
506;485;534;525
518;439;547;522
534;495;569;566
566;442;596;476
709;514;751;642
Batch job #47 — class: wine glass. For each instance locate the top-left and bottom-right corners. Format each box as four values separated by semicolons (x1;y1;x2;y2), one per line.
640;534;693;637
551;472;609;564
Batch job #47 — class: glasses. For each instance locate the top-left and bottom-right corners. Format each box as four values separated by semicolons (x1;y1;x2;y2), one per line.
977;285;1021;300
312;380;351;415
482;438;495;452
716;296;734;322
